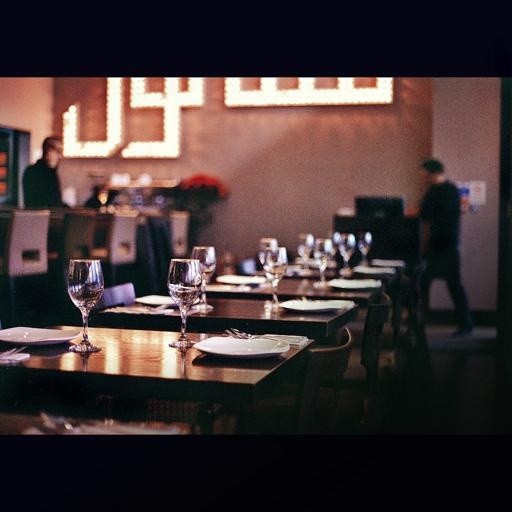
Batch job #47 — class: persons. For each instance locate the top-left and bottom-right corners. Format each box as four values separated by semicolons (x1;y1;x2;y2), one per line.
23;135;76;265
412;157;474;336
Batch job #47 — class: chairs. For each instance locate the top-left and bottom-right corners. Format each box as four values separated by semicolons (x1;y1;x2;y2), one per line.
1;204;191;324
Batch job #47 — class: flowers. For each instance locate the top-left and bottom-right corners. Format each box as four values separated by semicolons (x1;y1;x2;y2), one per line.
176;172;230;212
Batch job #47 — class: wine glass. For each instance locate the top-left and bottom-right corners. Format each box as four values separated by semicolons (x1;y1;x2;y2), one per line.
192;245;216;311
262;247;288;314
258;238;278;265
297;232;404;289
65;257;105;356
166;259;203;350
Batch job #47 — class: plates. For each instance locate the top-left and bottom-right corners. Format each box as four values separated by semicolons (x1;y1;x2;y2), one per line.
214;274;267;284
280;300;354;311
132;293;203;306
0;325;83;347
193;336;290;359
